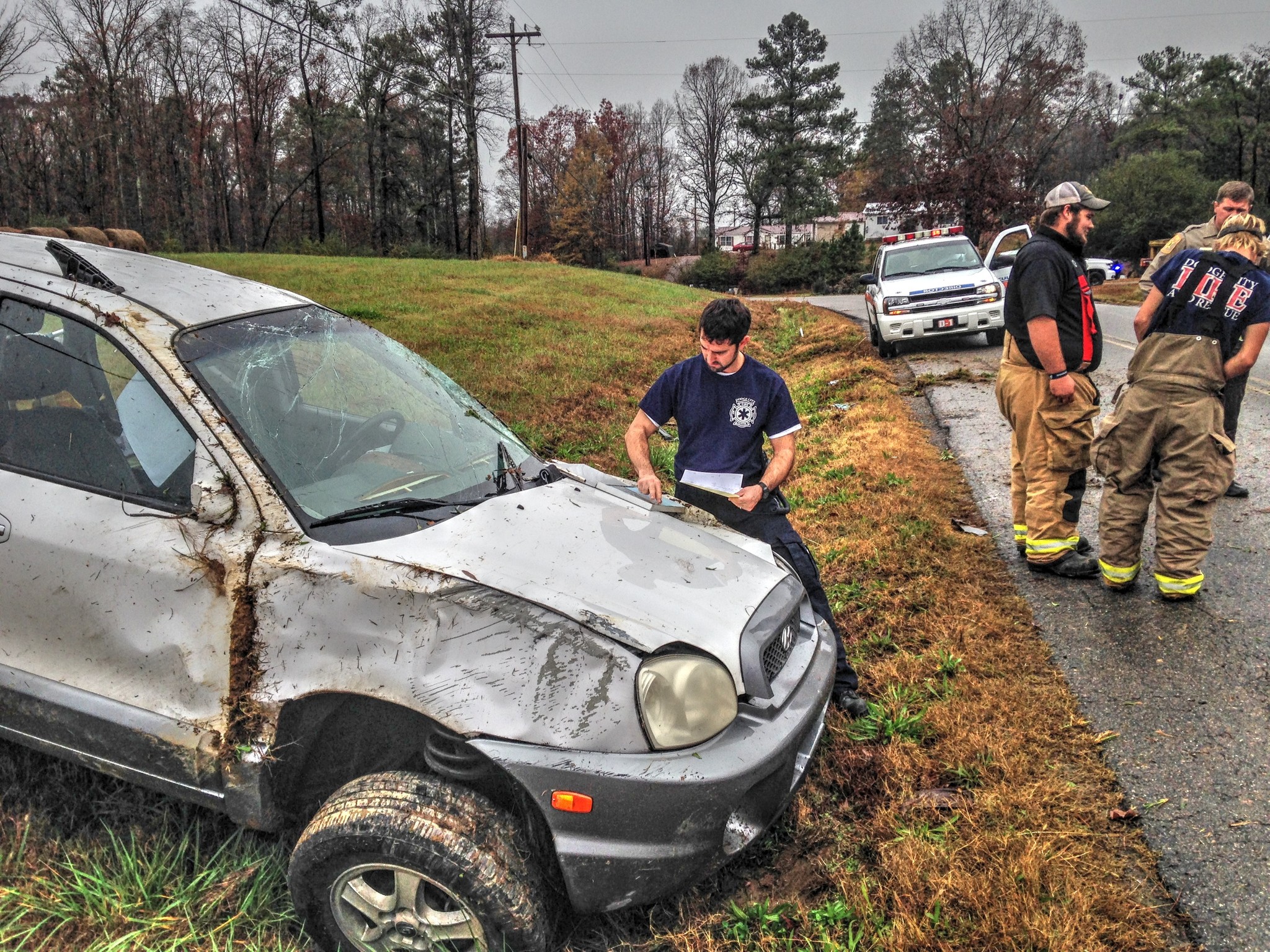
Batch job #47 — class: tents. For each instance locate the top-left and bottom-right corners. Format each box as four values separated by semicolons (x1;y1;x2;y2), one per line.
649;243;675;259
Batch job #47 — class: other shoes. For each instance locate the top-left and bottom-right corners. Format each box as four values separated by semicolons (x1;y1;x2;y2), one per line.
837;690;870;717
1225;480;1248;497
1032;550;1101;575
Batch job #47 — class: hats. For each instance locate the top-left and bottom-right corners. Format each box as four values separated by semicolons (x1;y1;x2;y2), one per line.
1043;180;1111;210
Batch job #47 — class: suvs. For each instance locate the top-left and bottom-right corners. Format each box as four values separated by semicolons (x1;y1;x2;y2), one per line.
859;223;1032;358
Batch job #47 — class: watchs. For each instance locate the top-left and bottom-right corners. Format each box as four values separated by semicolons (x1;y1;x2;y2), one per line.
758;482;770;500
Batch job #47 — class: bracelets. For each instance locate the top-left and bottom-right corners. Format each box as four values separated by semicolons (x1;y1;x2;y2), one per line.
1048;370;1068;379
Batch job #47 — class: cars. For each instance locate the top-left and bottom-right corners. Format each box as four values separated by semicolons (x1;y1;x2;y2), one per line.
997;249;1116;286
731;241;763;252
0;231;843;951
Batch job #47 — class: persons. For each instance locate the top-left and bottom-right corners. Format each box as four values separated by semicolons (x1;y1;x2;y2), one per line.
624;298;868;719
1088;211;1269;604
995;180;1112;578
1139;181;1258;497
